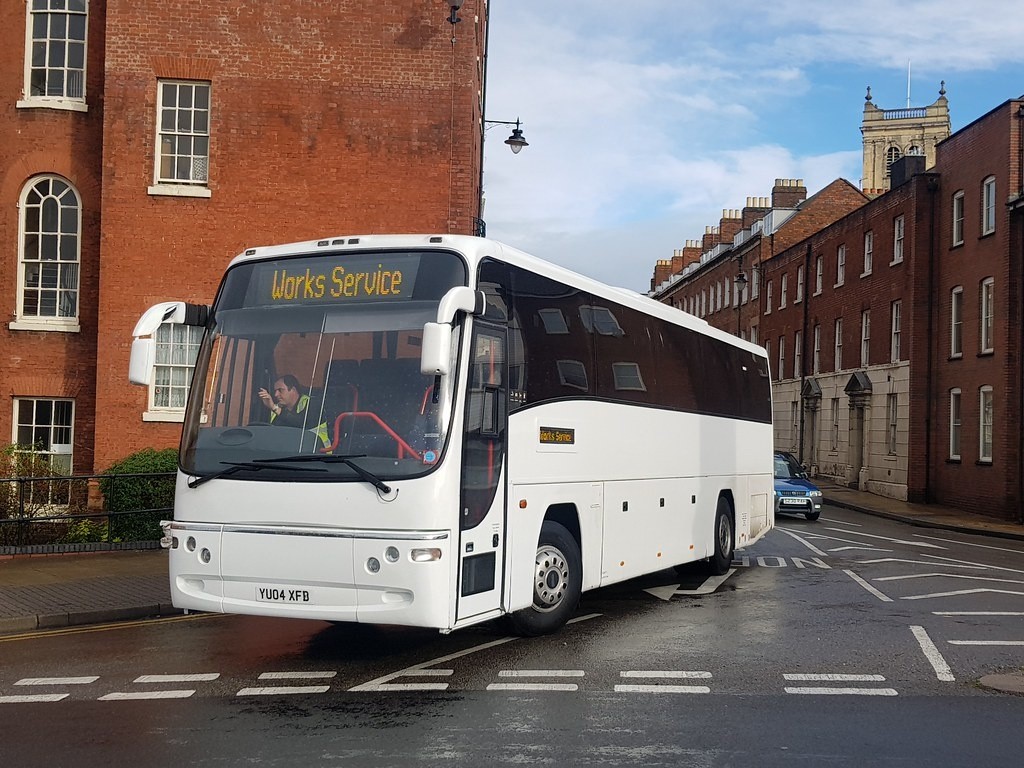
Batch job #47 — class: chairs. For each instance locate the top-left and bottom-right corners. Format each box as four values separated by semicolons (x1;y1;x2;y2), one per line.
321;359;358;414
360;358;394;420
394;358;430;418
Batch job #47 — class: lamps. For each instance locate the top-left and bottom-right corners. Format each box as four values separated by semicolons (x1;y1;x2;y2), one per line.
484;118;531;155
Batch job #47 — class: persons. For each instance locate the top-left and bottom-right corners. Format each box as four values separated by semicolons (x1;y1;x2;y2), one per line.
258;375;332;455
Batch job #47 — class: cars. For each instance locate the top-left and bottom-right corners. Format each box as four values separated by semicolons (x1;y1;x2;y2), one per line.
774;448;824;523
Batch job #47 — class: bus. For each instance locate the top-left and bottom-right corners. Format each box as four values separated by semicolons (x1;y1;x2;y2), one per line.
126;232;777;638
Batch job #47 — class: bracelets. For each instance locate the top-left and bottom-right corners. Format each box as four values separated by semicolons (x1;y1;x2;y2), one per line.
272;404;278;412
